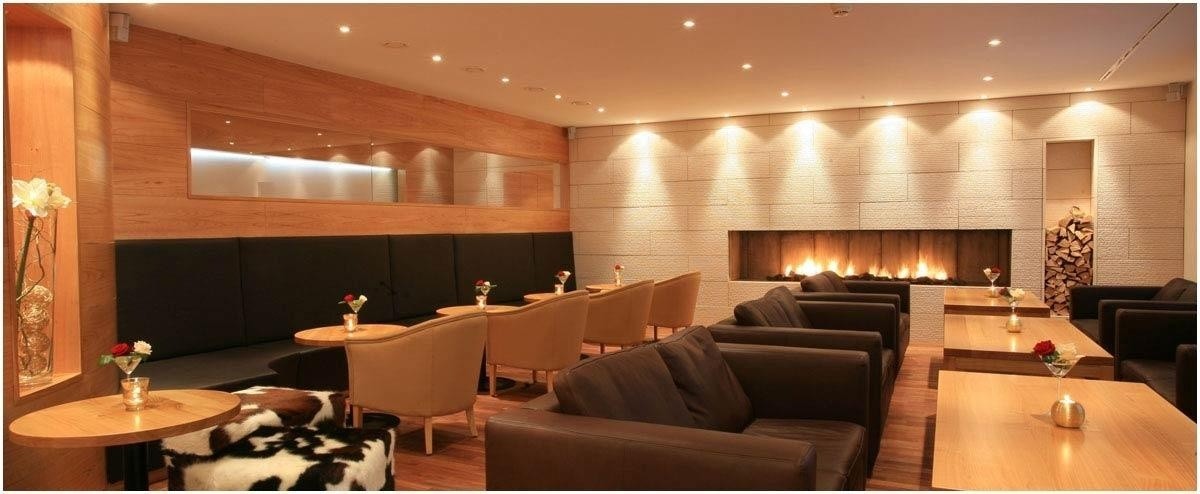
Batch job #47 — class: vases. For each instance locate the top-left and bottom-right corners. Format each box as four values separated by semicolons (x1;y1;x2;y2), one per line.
13;209;57;387
986;274;1000;287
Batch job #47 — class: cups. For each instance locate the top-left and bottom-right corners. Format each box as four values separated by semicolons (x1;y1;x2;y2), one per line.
615;277;624;288
342;313;358;333
120;376;151;412
475;295;488;311
554;283;564;295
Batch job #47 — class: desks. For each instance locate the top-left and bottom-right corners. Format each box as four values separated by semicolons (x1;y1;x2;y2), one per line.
8;390;240;491
931;287;1197;491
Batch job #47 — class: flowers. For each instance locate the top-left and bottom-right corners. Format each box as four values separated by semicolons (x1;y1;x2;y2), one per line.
98;341;153;365
12;177;71;307
614;265;624;272
982;267;1001;274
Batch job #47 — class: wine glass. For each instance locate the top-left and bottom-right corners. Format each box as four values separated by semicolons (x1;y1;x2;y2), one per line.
613;269;626;278
111;354;144;379
557;275;570;284
347;300;367;314
999;288;1028;328
1037;350;1082;418
983;269;1001;293
479;285;493;296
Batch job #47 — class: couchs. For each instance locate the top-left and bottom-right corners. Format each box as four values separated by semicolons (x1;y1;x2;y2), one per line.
1070;278;1197;350
